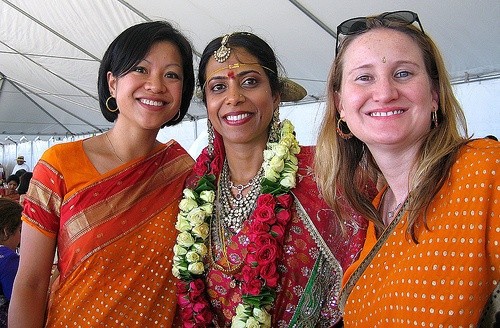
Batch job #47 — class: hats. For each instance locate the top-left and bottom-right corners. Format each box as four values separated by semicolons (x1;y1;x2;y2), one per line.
15;156;25;162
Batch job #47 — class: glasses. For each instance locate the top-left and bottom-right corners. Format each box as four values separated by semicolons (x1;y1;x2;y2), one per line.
335;10;427;58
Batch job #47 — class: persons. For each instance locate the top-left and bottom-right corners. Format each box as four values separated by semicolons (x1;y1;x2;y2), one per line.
7;20;196;327
315;14;500;327
198;32;384;327
0;154;34;314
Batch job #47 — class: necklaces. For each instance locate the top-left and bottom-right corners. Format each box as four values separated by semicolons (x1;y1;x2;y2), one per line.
207;157;265;273
388;202;404;218
377;187;389;212
175;118;299;326
105;132;125;164
